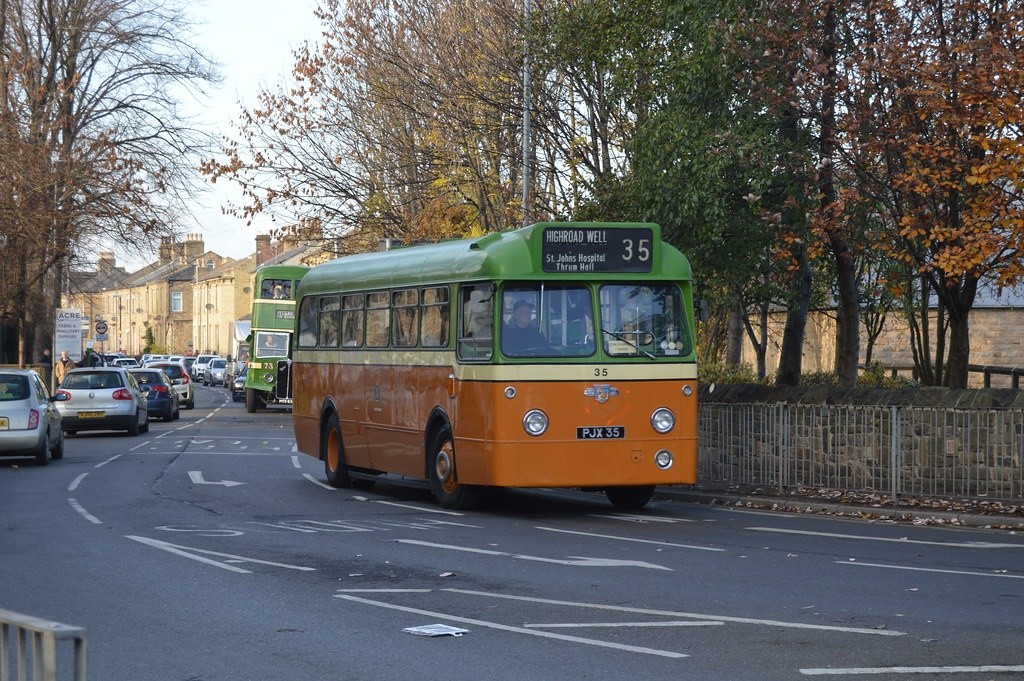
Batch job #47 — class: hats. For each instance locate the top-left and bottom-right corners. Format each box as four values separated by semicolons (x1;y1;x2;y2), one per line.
511;301;534;311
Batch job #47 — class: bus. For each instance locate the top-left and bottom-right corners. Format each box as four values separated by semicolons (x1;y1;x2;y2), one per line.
294;221;699;509
245;264;313;413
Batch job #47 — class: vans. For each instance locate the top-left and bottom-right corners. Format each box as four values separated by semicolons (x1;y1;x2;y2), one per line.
227;342;249;389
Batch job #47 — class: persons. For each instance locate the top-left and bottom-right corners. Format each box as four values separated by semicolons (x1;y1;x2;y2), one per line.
56;351;76;386
273;285;291;299
40;348;51;363
241;351;249;359
503;300;548;353
92;348;107;367
185;350;193;356
263;336;279;348
194;350;199;357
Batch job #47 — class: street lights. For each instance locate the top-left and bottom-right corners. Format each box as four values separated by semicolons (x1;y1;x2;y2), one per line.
114;294;122;352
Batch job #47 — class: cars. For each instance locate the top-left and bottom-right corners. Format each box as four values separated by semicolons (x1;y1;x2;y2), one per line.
102;353;139;369
144;363;196;409
127;369;181;421
232;366;247;401
52;367;150;435
140;355;228;387
0;367;68;467
